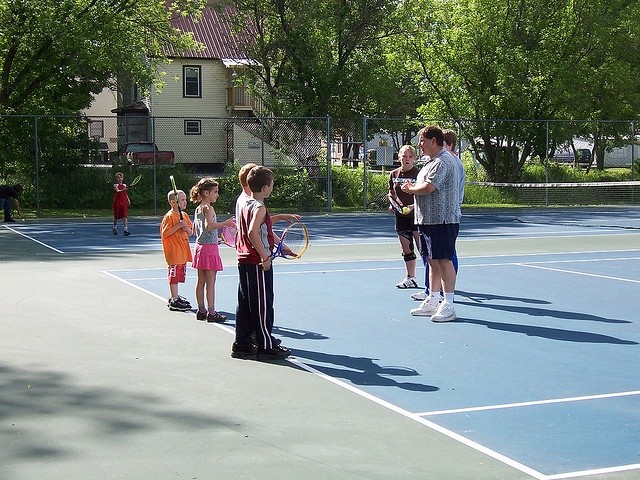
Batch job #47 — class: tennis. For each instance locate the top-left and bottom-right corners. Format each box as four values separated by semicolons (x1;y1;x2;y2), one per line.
402;207;409;214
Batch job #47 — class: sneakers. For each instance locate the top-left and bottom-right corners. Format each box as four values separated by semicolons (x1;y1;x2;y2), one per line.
167;295;190;307
250;330;281;346
169;297;192;312
124;231;131;236
256;345;292;361
196;309;207;320
410;296;440;316
231;341;258;360
395;274;418;289
410;290;429;300
113;229;117;235
430;300;457;322
4;218;16;222
208;311;227;322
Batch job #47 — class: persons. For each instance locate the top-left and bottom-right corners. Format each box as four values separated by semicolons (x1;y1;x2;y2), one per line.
1;182;15;222
388;145;421;290
400;126;461;323
232;167;296;362
160;189;193;311
112;172;131;236
189;178;236;323
236;165;301;261
412;129;464;303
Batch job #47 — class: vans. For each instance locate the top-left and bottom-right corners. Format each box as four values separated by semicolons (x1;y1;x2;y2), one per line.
553;149;591;168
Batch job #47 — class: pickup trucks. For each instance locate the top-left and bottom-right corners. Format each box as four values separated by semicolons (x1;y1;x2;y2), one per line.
112;142;174;167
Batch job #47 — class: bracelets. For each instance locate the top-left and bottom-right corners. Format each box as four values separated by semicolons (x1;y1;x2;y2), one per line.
261;256;271;262
